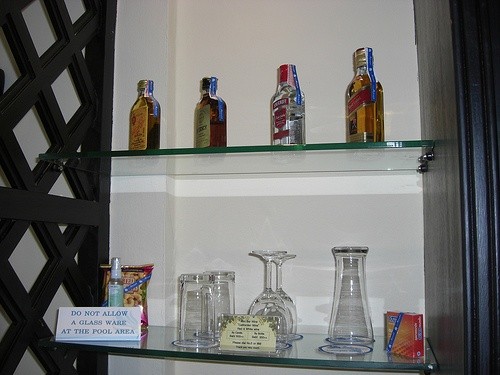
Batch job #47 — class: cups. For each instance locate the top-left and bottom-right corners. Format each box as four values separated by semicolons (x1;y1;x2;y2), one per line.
179;274;216;346
326;247;373;345
200;271;236;334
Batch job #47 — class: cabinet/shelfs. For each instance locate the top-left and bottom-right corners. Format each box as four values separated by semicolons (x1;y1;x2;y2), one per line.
45;141;439;375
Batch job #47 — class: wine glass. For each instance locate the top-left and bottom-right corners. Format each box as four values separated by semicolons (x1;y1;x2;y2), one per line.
248;250;291;350
272;254;297;339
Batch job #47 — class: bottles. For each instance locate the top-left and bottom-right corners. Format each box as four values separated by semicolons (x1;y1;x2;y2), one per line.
193;76;227;148
129;80;161;150
271;64;306;145
106;257;124;307
345;47;384;144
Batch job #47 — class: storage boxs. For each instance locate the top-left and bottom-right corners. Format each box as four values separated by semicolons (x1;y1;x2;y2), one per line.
385;311;425;358
387;352;426;365
